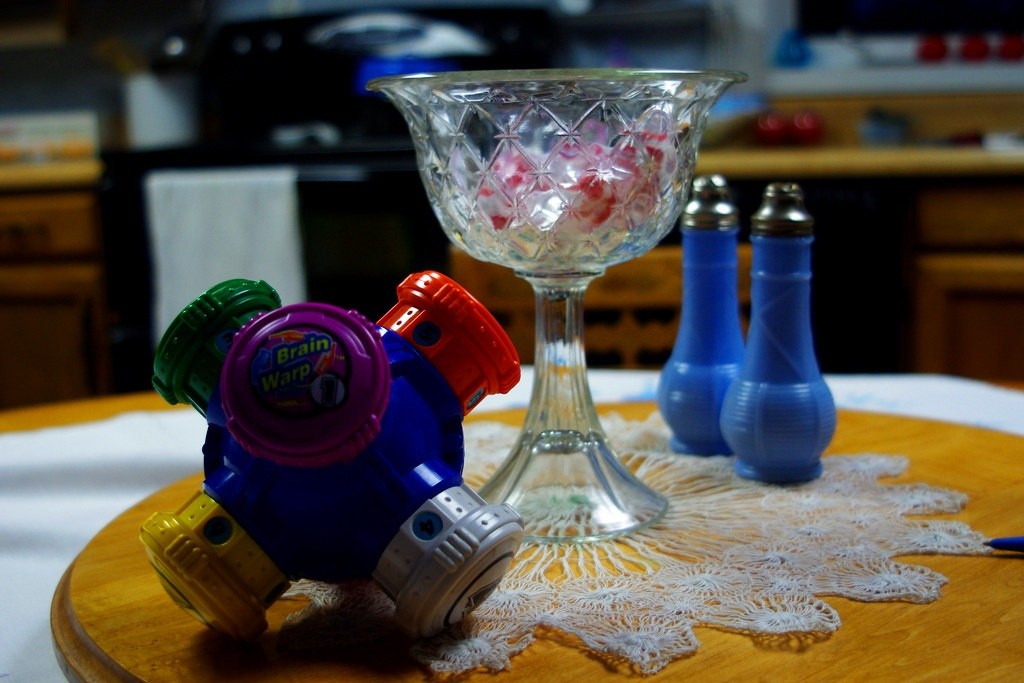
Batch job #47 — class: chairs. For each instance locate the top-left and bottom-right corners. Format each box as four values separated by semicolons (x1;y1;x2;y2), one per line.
452;241;751;370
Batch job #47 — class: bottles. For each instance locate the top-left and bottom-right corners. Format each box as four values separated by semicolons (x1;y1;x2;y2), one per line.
653;174;746;457
720;184;837;485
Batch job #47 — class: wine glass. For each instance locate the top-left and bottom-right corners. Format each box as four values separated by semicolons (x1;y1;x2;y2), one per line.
371;66;747;543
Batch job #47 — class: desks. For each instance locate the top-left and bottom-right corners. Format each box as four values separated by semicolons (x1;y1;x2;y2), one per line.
0;374;1024;683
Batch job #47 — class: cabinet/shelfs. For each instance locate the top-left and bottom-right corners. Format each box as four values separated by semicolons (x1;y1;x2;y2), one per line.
684;172;1024;387
0;192;114;415
